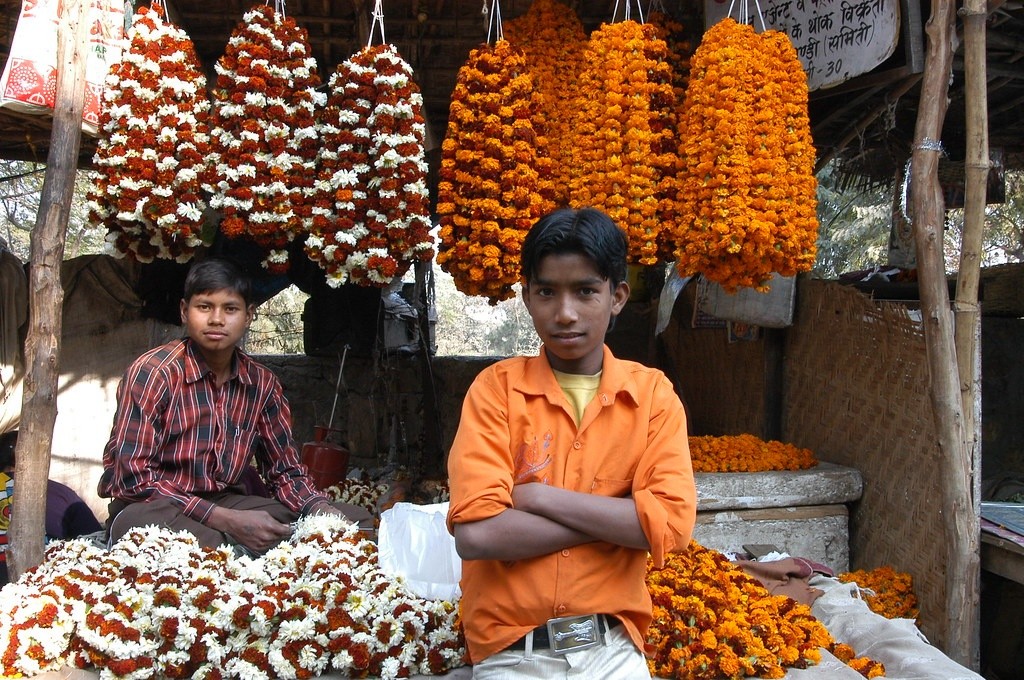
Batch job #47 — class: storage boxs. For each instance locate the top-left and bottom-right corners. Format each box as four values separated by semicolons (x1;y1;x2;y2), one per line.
692;462;862;577
379;500;462;601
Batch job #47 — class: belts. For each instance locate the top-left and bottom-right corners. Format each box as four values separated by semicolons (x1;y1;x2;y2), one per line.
503;613;620;656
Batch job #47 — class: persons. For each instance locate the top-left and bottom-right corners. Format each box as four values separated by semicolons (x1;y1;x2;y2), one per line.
0;431;104;541
446;208;697;680
97;261;375;549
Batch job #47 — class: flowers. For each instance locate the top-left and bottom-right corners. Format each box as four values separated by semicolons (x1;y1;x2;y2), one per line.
643;536;921;680
411;478;451;506
689;432;817;473
0;510;473;680
324;466;411;512
86;1;819;306
